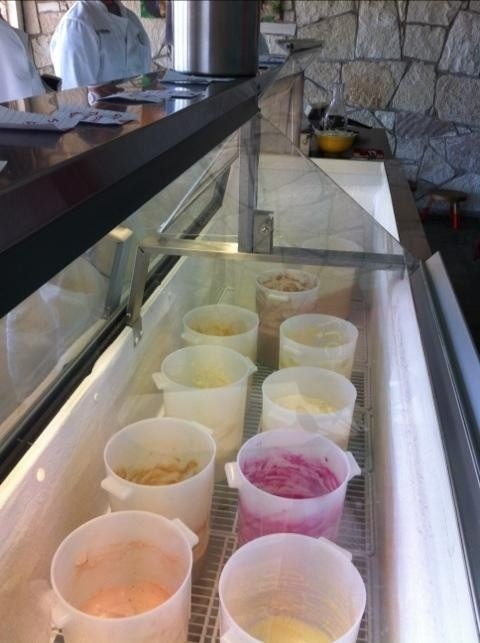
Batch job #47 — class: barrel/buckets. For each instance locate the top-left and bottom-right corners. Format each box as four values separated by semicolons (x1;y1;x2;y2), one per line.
259;365;357;465
216;533;365;641
222;432;360;555
253;266;323;362
178;298;262;377
152;343;257;486
50;510;200;642
100;417;213;558
273;314;359;385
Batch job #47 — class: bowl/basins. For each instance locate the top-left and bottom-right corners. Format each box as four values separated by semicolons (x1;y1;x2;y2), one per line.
314;130;356;153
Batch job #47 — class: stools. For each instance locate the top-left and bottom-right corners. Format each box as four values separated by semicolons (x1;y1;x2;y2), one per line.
420;186;471;230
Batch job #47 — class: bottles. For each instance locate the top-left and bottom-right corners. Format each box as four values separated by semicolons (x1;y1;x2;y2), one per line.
324;81;348;130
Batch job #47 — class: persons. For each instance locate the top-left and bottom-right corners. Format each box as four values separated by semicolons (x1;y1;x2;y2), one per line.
1;8;55;110
45;0;154;91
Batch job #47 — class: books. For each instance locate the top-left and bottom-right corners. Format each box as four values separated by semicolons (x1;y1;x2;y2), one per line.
258;52;291;70
